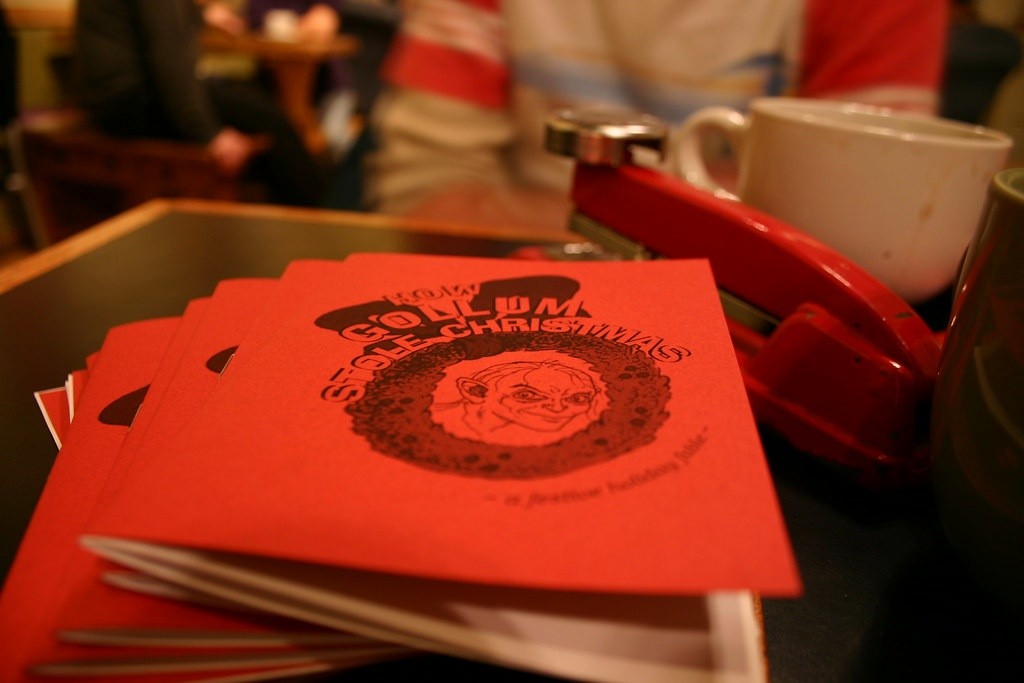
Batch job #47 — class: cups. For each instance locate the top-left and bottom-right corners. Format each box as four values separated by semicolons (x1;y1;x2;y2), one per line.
674;96;1014;302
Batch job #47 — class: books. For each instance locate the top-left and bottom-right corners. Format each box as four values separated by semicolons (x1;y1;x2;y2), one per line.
0;248;800;683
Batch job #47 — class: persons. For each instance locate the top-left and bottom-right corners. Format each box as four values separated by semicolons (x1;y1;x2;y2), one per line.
0;0;1024;234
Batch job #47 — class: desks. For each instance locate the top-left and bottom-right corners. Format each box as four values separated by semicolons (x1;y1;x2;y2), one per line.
0;199;916;683
